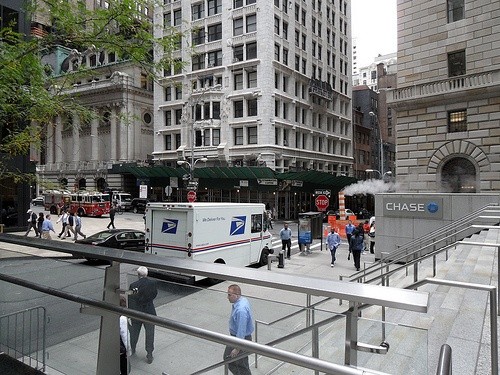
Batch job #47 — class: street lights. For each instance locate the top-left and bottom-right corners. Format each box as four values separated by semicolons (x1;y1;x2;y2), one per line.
364;111;392;180
177;88;211;182
91;134;108;181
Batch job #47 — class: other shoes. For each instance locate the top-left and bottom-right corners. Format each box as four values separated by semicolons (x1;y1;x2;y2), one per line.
58;235;61;238
331;264;334;267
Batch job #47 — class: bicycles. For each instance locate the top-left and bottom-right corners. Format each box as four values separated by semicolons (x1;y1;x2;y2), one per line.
115;205;124;215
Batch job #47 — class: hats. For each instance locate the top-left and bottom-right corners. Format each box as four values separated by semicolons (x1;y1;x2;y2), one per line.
27;209;32;214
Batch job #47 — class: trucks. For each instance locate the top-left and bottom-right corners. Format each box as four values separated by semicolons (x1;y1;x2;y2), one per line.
104;191;134;212
142;202;274;286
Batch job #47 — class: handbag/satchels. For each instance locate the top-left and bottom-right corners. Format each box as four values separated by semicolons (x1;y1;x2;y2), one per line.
348;252;350;260
368;232;375;238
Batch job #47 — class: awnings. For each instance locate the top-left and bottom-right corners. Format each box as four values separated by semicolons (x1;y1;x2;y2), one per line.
134;166;358;187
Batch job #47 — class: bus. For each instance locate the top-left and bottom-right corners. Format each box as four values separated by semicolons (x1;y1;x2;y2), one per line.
41;189;111;218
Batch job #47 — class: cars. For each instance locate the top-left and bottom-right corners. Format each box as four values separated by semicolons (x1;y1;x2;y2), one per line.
336;208;356;220
71;228;145;265
322;209;337;223
31;197;44;206
354;208;372;220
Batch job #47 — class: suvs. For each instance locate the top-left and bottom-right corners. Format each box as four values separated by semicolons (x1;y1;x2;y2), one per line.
130;198;150;214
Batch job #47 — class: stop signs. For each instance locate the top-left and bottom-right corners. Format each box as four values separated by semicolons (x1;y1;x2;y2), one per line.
186;191;197;202
315;195;329;209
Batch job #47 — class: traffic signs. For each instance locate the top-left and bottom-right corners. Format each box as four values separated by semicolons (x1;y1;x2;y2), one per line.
186;186;198;191
187;181;199;186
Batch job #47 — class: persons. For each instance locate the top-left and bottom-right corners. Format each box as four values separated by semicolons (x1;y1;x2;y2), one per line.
128;266;158;363
42;215;55;240
106;206;115;229
37;212;44;238
363;221;370;251
24;209;38;237
280;223;292;259
326;227;341;266
223;285;254;375
56;210;71;238
349;229;363;271
370;221;375;254
264;211;273;229
120;296;132;375
72;212;86;241
345;220;355;244
61;211;75;240
357;222;364;244
